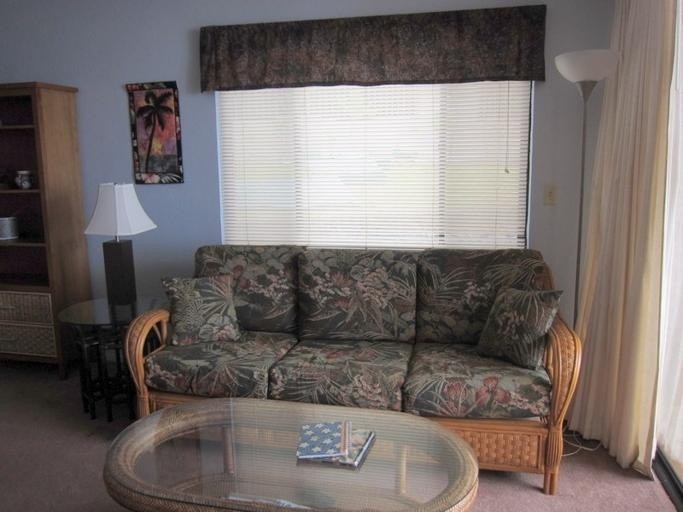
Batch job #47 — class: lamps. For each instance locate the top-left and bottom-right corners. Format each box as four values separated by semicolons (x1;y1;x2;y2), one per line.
553;49;613;329
82;183;157;304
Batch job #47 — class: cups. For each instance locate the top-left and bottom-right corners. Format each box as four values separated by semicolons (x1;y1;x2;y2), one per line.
0;217;18;240
16;171;32;189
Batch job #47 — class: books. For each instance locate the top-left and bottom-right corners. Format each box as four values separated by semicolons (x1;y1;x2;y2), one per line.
295;419;376;469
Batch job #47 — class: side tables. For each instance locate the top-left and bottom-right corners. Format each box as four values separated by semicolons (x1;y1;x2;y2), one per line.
57;299;165;422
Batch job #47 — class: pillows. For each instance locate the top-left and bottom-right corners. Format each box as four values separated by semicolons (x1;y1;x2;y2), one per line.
476;286;563;370
161;274;240;345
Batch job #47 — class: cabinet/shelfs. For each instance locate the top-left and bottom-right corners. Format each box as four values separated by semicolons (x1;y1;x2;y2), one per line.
1;82;96;380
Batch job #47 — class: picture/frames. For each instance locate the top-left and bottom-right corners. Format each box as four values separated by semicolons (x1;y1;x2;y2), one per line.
124;80;183;184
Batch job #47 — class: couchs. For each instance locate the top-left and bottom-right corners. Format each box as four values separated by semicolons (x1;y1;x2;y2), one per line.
125;245;581;495
